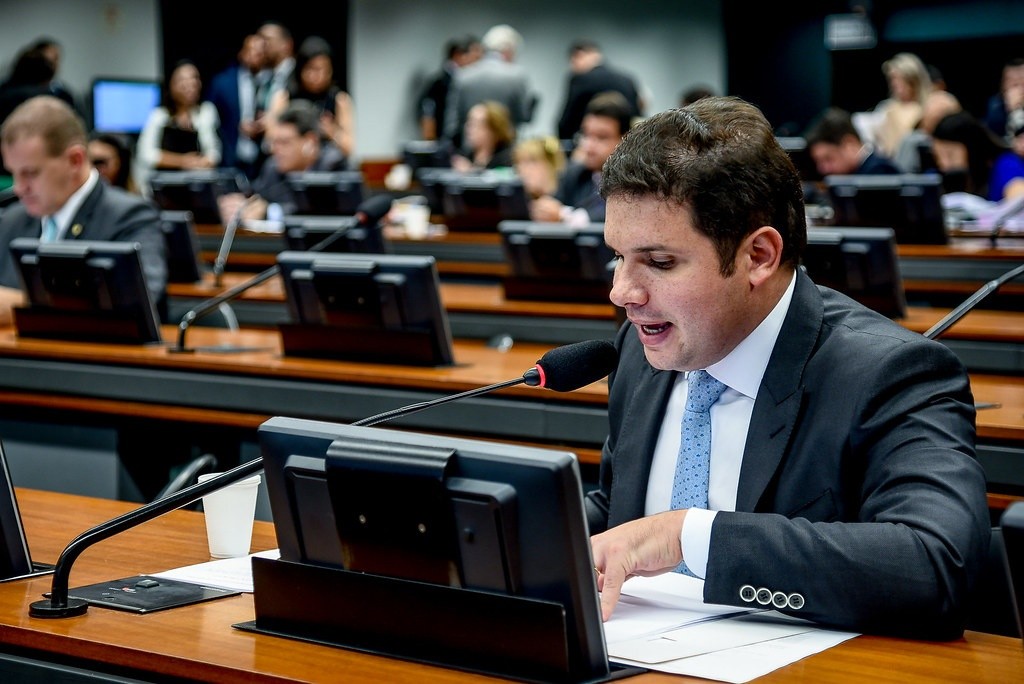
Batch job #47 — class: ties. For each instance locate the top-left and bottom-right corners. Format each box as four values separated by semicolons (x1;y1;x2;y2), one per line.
672;370;726;578
42;216;57;243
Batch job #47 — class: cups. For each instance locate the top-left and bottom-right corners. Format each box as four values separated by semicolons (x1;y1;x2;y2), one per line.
199;473;262;558
405;206;431;240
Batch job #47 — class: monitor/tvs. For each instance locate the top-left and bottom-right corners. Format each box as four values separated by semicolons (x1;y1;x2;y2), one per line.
0;75;950;684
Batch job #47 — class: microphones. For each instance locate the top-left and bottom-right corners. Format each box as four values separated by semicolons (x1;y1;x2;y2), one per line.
34;339;617;616
169;190;392;355
210;180;274;284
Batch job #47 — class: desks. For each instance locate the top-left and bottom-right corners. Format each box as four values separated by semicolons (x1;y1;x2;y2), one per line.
166;272;1024;383
0;486;1024;684
0;324;1024;511
193;220;1024;313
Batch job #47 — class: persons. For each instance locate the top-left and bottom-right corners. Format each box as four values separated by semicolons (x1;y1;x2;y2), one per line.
0;96;166;327
558;42;646;140
583;96;991;641
450;101;514;171
417;36;485;141
807;53;1024;200
679;84;714;106
216;98;361;225
443;25;539;143
510;134;565;199
0;24;355;200
532;91;633;220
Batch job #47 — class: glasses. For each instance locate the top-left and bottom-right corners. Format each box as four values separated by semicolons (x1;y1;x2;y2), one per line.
94;157;117;169
